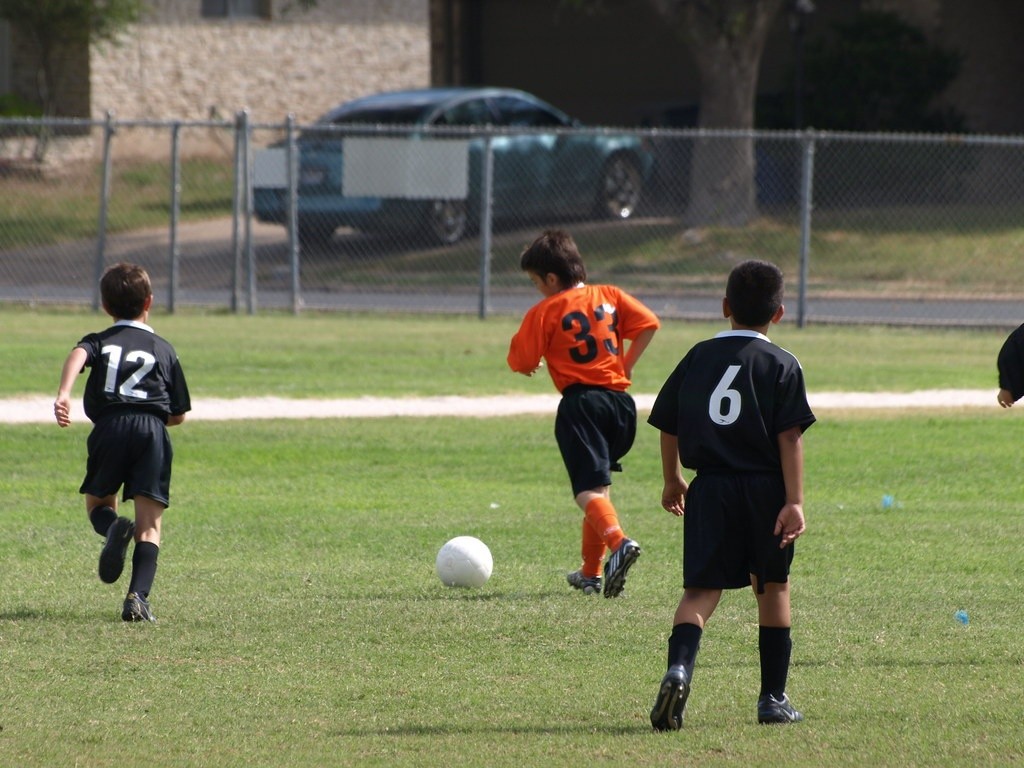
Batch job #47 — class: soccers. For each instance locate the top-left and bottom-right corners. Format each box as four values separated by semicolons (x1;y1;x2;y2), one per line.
434;535;496;590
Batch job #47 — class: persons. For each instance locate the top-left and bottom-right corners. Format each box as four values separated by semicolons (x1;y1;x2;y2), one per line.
646;260;817;733
998;322;1024;408
54;259;191;624
506;230;659;597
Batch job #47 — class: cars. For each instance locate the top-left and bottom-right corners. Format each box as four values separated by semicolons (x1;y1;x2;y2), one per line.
243;88;653;245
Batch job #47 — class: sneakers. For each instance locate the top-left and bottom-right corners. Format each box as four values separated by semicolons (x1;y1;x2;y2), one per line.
567;569;603;595
758;691;804;724
650;663;690;730
121;591;157;623
99;516;136;583
603;537;641;598
